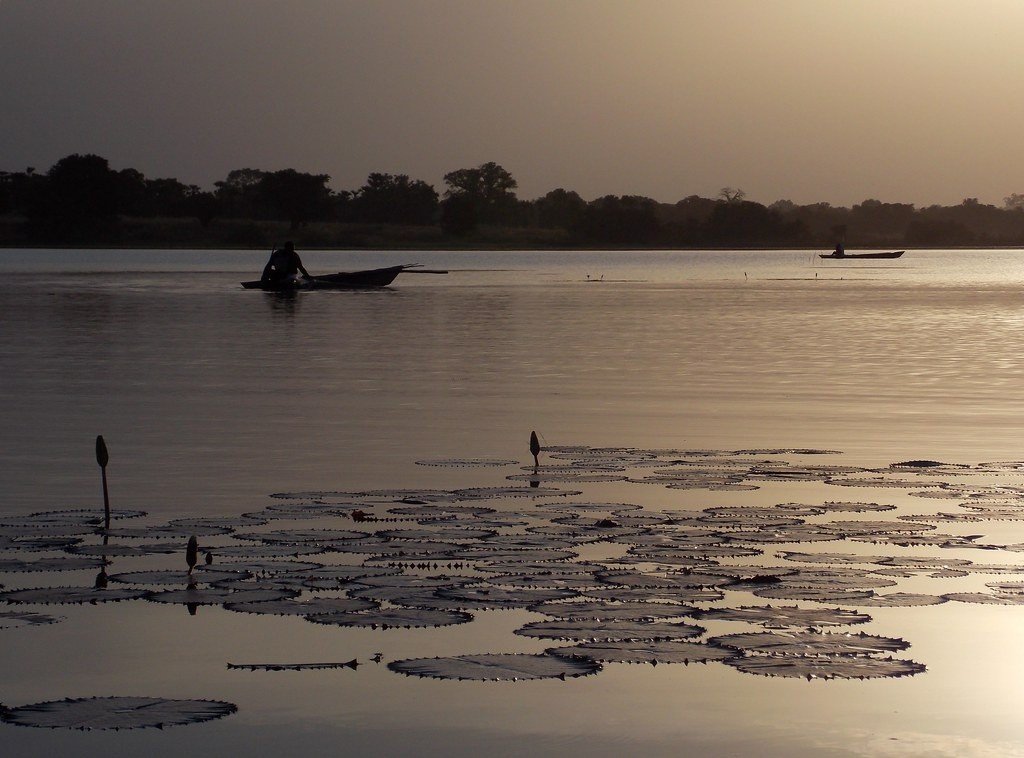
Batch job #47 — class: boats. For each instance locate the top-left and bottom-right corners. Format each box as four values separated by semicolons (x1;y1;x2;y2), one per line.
819;251;906;259
240;263;402;289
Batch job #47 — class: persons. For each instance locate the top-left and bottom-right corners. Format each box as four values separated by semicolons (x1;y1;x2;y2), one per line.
831;244;844;255
262;240;312;280
